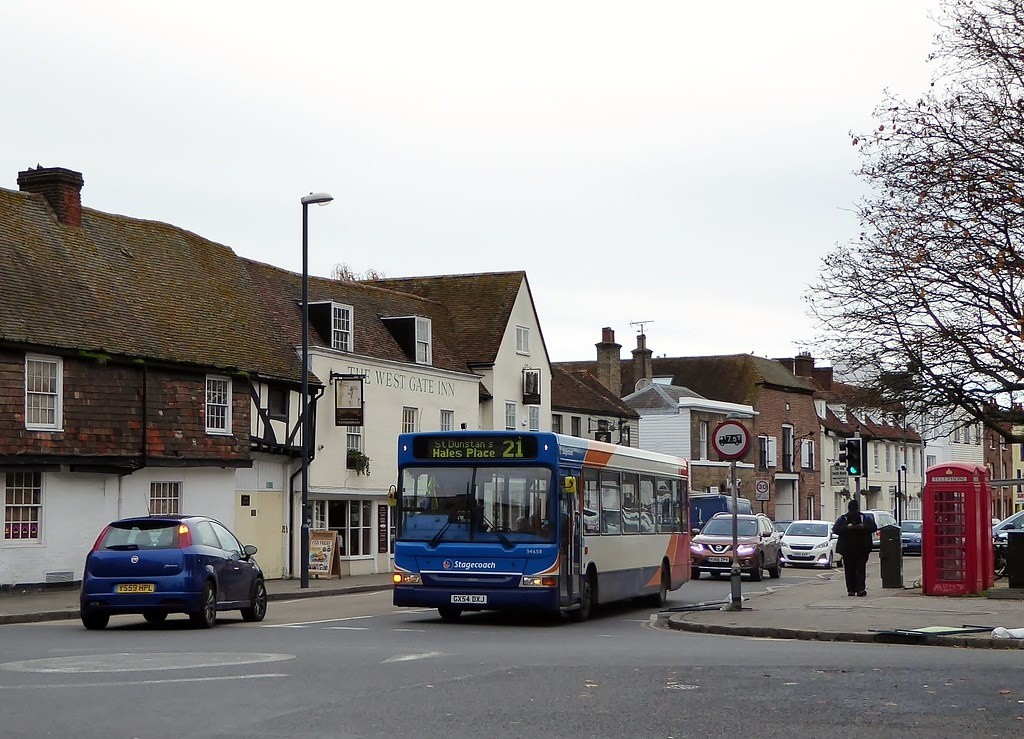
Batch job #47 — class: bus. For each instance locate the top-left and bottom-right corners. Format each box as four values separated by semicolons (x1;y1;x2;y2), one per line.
386;427;693;623
688;491;753;533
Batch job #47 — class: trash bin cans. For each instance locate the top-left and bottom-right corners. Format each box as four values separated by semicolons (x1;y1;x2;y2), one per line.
1006;529;1024;589
877;524;903;588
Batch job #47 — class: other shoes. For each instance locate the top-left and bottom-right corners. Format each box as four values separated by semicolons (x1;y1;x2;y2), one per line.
848;591;855;596
857;591;868;596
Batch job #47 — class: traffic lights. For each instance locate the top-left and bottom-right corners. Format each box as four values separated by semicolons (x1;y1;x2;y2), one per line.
845;436;863;478
837;440;846;466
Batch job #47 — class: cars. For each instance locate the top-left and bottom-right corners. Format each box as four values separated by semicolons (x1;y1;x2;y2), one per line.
79;513;269;630
777;519;844;570
898;519;923;556
773;519;793;539
993;509;1024;543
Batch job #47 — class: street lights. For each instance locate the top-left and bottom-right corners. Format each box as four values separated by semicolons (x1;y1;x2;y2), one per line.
901;465;909;519
296;189;338;589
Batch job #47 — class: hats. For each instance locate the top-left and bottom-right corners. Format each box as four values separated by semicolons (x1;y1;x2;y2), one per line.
849;500;858;509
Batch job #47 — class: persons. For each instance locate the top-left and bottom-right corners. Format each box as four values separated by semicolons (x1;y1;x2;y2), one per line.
516;515;536;532
833;500;877;596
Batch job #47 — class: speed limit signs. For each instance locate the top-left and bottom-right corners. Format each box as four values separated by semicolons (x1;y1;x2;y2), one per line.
755;479;770;501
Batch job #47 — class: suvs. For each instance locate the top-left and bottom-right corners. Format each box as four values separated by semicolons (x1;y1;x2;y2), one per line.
691;510;782;581
862;508;897;549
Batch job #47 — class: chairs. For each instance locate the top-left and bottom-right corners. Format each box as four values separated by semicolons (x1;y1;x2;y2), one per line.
135;532;151;546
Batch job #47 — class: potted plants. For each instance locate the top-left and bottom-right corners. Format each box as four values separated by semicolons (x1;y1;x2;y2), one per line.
347;449;373;478
